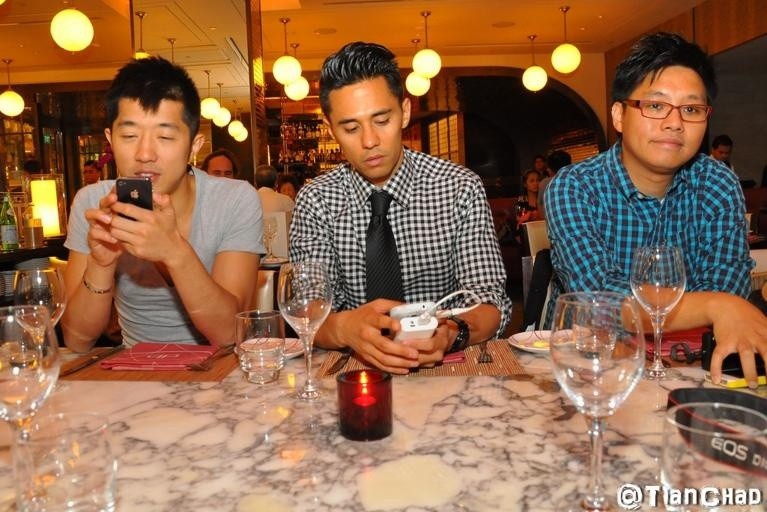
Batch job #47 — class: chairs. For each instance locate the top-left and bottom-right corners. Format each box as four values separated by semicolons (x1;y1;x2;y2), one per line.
250;259;285;338
47;258;74;313
520;220;553;333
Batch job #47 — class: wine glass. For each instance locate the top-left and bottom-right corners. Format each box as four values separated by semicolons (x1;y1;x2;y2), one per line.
10;265;67;345
1;305;65;512
661;401;766;512
628;244;687;380
274;259;333;400
548;289;647;512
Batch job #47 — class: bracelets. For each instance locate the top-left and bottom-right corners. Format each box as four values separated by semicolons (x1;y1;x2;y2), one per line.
79;274;113;294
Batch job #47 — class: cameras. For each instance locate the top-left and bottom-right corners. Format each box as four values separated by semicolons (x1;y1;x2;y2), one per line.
699;332;764;373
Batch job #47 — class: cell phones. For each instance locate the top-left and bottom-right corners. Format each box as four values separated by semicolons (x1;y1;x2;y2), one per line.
388;302;435;337
115;177;153;221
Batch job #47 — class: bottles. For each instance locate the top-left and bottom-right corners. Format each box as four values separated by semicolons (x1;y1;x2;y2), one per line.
22;218;43;249
278;147;340;164
0;190;19;252
277;121;326;139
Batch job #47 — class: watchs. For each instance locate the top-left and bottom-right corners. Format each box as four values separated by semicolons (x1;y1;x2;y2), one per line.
449;317;470;356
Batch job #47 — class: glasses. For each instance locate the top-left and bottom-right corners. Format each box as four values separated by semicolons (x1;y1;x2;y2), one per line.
619;99;712;124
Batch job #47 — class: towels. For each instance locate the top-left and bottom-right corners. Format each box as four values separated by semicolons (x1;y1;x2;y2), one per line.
443;348;466;365
630;323;716;361
100;342;221;371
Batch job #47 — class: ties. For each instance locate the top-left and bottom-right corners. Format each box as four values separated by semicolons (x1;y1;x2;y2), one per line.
363;193;406;336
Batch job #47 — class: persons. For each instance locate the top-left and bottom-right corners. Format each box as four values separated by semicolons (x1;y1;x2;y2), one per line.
199;147;298;234
710;134;735;173
515;149;572;330
23;158;45;175
61;57;269;354
288;41;513;374
543;33;767;390
81;159;103;186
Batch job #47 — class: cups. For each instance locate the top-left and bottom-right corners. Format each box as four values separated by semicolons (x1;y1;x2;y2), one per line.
16;409;120;512
232;308;285;380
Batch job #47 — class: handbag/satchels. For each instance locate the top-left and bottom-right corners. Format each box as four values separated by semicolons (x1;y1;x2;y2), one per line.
495;220;516;247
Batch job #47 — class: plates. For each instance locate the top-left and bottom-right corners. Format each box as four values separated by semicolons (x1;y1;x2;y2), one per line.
508;329;574;357
232;336;304;360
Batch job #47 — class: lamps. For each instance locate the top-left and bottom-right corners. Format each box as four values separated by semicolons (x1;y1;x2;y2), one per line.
228;100;244;137
49;1;95;56
200;70;220;120
0;57;26;117
212;82;231;127
522;34;548;92
133;10;149;59
283;41;309;102
411;10;441;79
233;112;248;142
272;16;302;84
550;4;580;74
29;177;61;239
404;38;431;97
